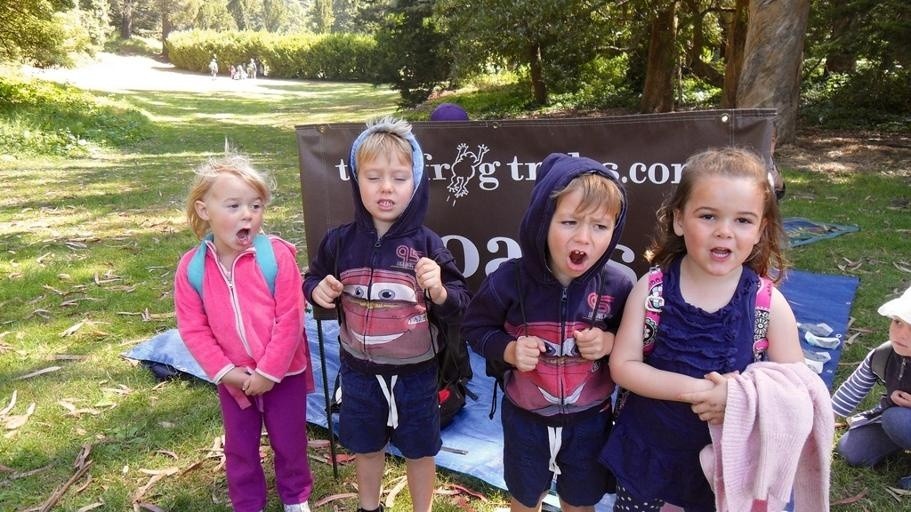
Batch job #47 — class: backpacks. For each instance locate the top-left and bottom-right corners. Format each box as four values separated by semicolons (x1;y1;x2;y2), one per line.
329;300;478;431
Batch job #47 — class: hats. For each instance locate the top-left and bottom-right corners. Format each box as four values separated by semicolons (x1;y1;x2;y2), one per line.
876;287;911;324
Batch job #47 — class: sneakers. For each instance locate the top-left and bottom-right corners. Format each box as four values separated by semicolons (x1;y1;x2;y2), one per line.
284;501;310;512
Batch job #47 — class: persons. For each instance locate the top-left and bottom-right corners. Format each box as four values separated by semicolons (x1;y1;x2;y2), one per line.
229;58;258;79
302;114;471;512
209;59;220;78
429;102;467;121
172;148;316;512
458;151;636;512
828;284;911;494
596;148;805;512
